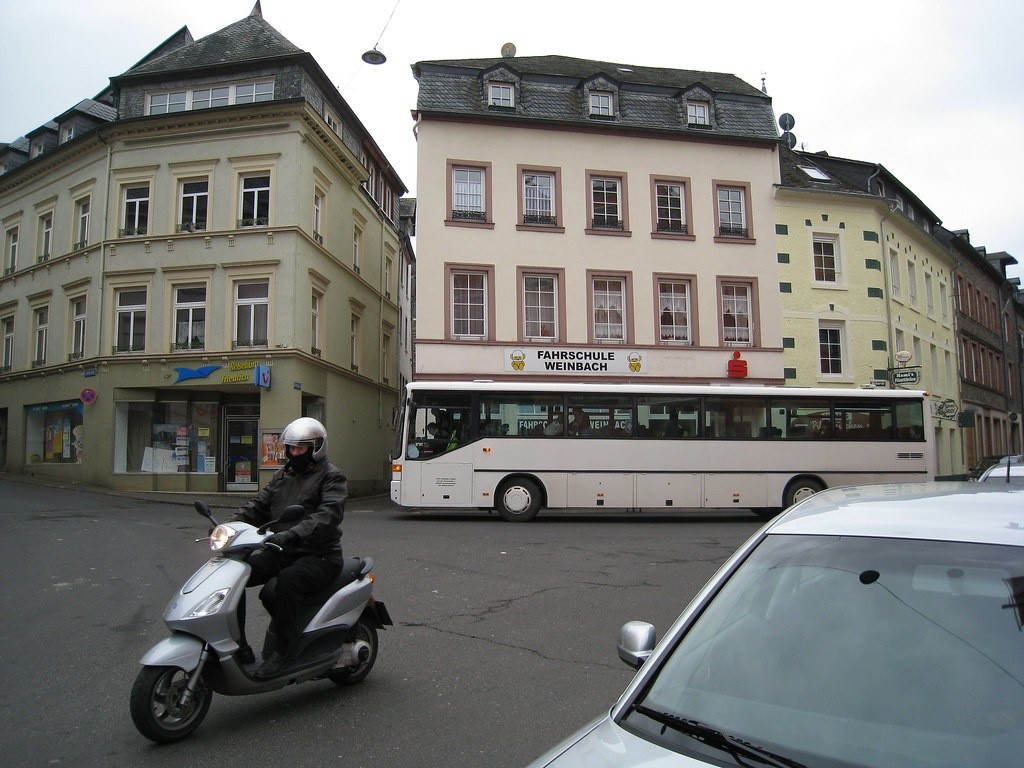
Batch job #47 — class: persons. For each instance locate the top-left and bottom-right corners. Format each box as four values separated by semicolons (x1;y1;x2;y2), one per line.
569;407;590;436
208;416;349;679
909;425;921;440
816;420;831;439
429;407;449;431
543;413;563;436
421;422;448;455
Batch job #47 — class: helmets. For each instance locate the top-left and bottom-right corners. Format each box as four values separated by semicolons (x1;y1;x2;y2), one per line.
281;417;328;462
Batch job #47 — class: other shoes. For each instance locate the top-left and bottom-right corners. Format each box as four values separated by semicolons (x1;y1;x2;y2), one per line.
255;652;290;678
233;645;255;664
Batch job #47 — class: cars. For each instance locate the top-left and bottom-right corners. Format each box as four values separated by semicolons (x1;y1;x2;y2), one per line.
524;479;1024;768
977;461;1024;483
967;455;1005;483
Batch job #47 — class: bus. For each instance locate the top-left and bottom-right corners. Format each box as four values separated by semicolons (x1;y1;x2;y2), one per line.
388;380;940;523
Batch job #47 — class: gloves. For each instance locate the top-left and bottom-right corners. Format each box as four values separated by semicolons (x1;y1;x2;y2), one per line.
269;529;301;552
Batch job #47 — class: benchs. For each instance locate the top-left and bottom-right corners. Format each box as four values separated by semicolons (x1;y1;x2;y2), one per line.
590;424;924;440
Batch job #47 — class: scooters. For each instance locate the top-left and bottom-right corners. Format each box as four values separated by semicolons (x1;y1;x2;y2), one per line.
129;500;394;746
